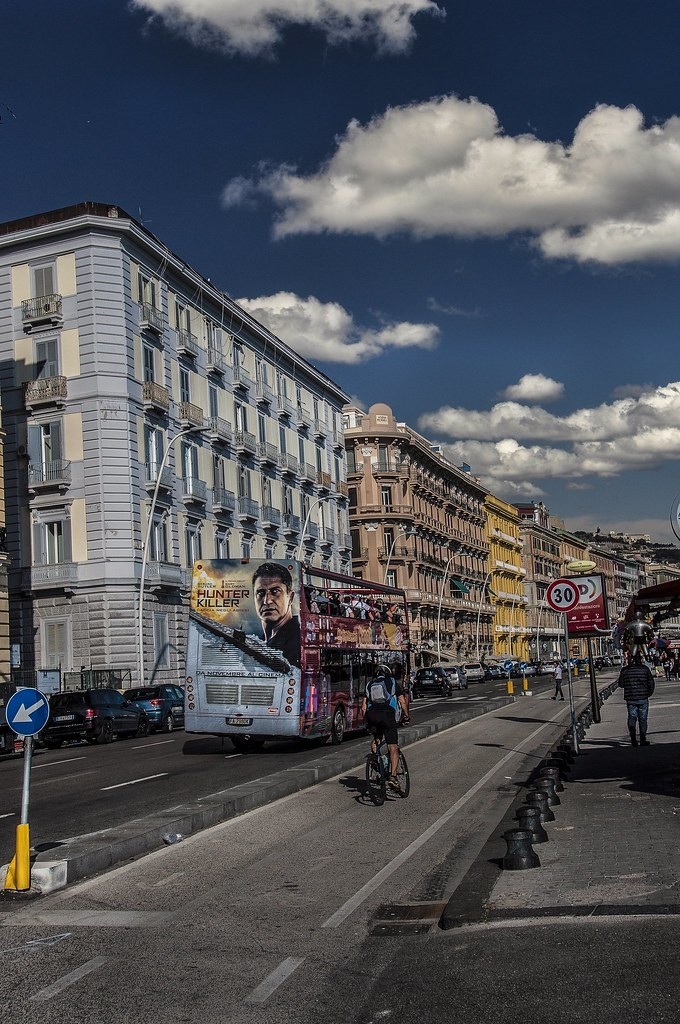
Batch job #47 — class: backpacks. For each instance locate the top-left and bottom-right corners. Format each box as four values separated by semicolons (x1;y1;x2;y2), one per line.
370;680;392;704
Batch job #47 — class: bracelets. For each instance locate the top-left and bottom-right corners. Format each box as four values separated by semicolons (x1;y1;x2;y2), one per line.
404;715;408;718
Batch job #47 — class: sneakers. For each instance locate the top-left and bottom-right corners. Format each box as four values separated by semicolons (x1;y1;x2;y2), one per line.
387;777;401;789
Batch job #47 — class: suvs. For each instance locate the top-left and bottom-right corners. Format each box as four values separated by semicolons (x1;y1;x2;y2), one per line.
413;667;453;698
462;663;486;684
43;688;150;748
444;665;469;690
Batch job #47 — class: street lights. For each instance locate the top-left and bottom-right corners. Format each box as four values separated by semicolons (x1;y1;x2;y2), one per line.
137;427;214;685
510;580;534;659
476;568;505;661
383;531;419;585
437;554;469;662
297;495;344;560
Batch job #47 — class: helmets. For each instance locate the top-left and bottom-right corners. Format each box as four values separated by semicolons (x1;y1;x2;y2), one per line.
375;664;391;674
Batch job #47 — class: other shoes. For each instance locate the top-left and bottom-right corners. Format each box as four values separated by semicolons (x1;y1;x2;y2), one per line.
551;696;556;700
631;742;639;747
558;698;565;701
641;741;651;747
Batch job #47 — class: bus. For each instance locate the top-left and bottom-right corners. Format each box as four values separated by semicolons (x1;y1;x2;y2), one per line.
183;559;421;749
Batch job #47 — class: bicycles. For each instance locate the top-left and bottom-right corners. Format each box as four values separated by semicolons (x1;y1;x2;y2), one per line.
365;715;411;807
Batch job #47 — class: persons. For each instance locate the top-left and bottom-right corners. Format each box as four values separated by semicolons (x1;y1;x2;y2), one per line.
361;666;410;789
619;657;655;746
624;612;653;662
252;563;301;669
627;651;680;681
307;583;399;622
549;661;564;701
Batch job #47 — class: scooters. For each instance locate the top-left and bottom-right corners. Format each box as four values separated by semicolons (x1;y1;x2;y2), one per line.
596;659;602;671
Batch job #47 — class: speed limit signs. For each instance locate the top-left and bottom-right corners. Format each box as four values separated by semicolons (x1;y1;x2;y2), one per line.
547;579;580;613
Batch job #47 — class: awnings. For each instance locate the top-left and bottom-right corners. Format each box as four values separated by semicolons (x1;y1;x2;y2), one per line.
486;586;498;597
451;578;470;593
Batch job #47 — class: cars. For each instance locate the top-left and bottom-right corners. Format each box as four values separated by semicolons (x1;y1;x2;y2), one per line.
537;660;555;674
122;682;184;734
603;655;624;666
516;663;536;676
488;665;507;679
559;660;575;673
